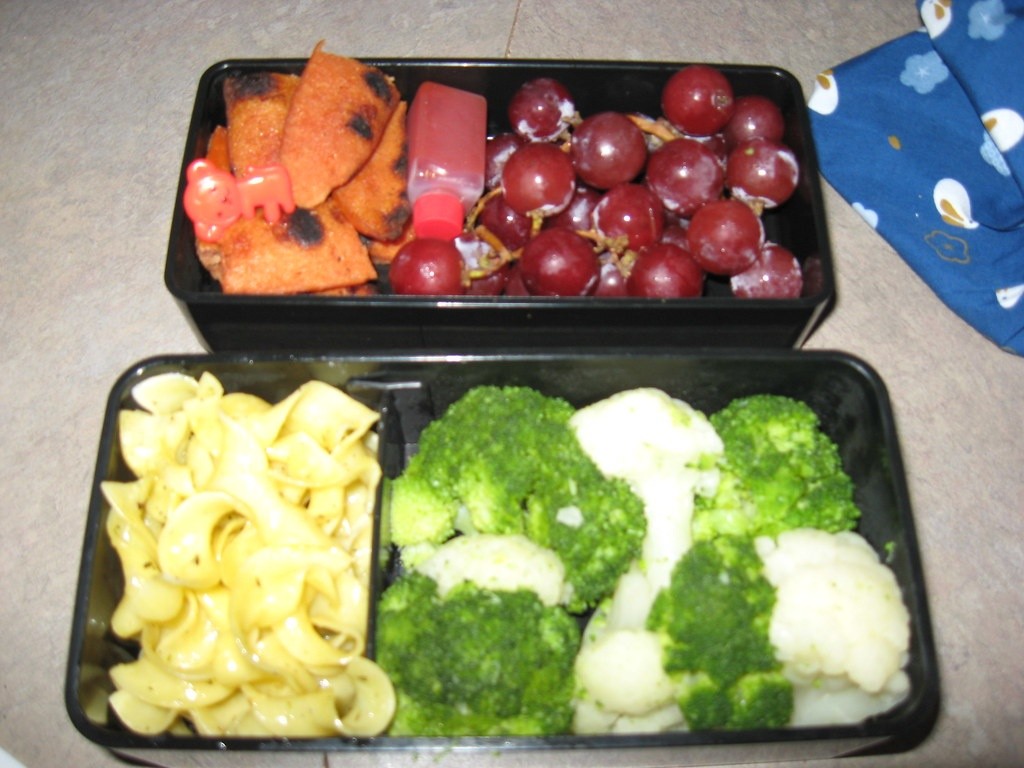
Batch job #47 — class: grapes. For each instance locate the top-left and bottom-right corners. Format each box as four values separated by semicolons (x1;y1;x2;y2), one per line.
389;63;805;301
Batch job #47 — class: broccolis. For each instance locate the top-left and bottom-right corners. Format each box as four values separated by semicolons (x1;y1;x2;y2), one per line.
375;383;913;738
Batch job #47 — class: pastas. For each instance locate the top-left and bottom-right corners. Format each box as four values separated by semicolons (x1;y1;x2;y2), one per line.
98;367;399;741
